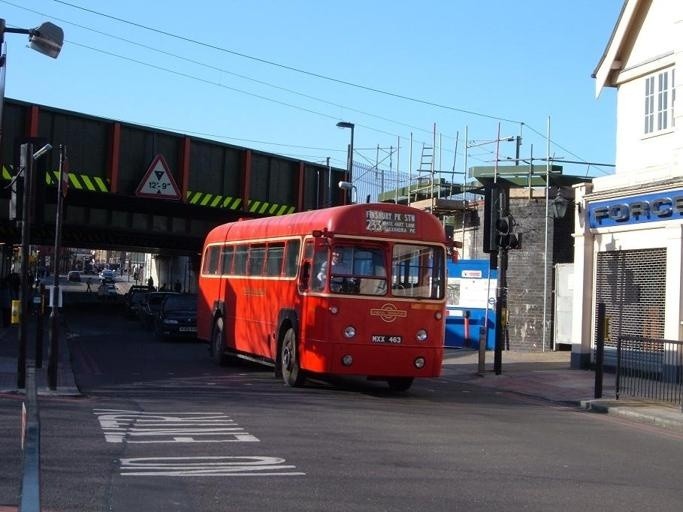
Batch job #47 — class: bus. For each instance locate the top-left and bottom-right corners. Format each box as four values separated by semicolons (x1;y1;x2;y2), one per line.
197;199;445;392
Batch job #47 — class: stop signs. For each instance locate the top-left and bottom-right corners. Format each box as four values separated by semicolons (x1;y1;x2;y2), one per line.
60;158;69;197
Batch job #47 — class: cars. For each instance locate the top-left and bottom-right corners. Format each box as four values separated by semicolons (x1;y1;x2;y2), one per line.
123;285;197;339
67;270;81;282
97;268;117;296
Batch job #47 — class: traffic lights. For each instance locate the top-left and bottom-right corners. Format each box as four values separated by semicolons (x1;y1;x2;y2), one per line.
497;214;511;235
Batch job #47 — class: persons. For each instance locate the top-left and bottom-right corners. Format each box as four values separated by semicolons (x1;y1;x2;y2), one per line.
317;246;354;290
86;278;93;293
176;279;182;293
147;276;153;286
158;283;167;292
